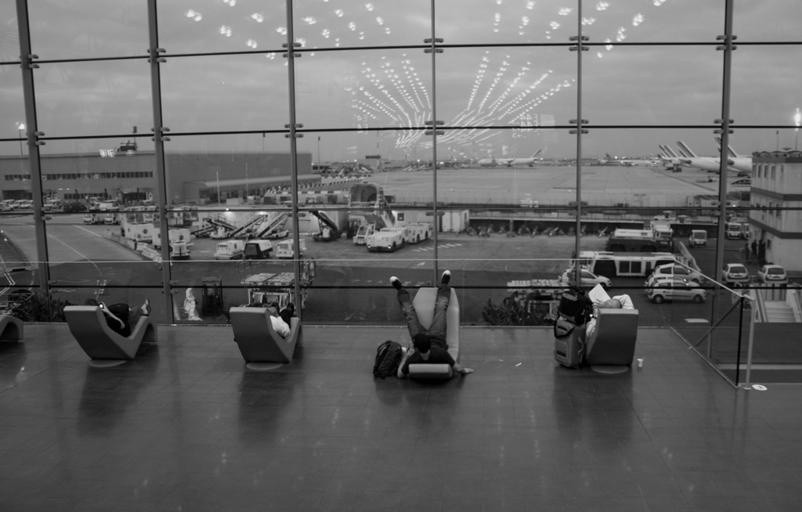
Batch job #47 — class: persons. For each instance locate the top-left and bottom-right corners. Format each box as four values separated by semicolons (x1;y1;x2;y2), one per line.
577;285;623;338
744;235;765;262
84;296;152;337
388;270;475;380
239;300;295;340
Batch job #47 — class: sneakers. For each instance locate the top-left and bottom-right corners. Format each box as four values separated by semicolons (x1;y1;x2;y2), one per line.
390;276;402;290
441;269;451;284
287;303;294;316
141;298;150;315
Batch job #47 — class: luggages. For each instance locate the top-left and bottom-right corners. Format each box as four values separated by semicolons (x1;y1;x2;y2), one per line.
555;319;585;368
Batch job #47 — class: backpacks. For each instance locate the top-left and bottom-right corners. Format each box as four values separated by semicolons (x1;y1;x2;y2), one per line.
373;340;401;380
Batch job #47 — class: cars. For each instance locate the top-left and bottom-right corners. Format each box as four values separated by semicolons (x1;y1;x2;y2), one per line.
759;265;788;287
644;264;705;302
688;230;708;247
723;263;751;287
742;223;750;240
559;269;612;290
726;222;743;240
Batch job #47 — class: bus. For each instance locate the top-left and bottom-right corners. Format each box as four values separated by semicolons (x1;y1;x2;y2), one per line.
572;250;686;278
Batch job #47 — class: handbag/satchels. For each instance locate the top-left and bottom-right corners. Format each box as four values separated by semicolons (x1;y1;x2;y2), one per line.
559;287;589;325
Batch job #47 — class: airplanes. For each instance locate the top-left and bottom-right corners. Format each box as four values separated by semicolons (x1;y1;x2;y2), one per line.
599;152;652;167
650;135;752;183
478;149;542;169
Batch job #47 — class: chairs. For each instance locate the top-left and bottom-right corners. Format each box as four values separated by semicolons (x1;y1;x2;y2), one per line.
408;288;460;377
587;309;640;375
229;307;303;372
0;314;25;344
63;305;158;368
